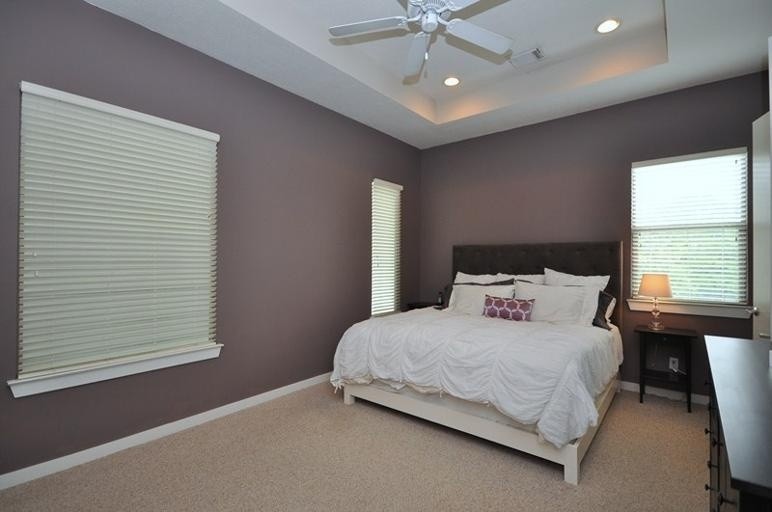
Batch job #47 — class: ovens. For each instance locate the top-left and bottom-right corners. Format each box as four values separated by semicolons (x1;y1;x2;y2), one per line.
328;240;625;485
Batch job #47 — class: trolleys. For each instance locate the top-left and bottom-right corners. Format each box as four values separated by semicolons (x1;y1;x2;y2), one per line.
328;0;514;79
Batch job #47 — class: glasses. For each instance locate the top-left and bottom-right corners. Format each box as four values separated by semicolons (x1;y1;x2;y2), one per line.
637;274;673;331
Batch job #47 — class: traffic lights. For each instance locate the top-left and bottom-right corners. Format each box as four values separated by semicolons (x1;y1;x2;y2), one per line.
544;268;611;328
449;284;515;316
482;294;536;322
514;281;587;326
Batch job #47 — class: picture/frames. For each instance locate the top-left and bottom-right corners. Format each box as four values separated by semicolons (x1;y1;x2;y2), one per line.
703;334;772;512
